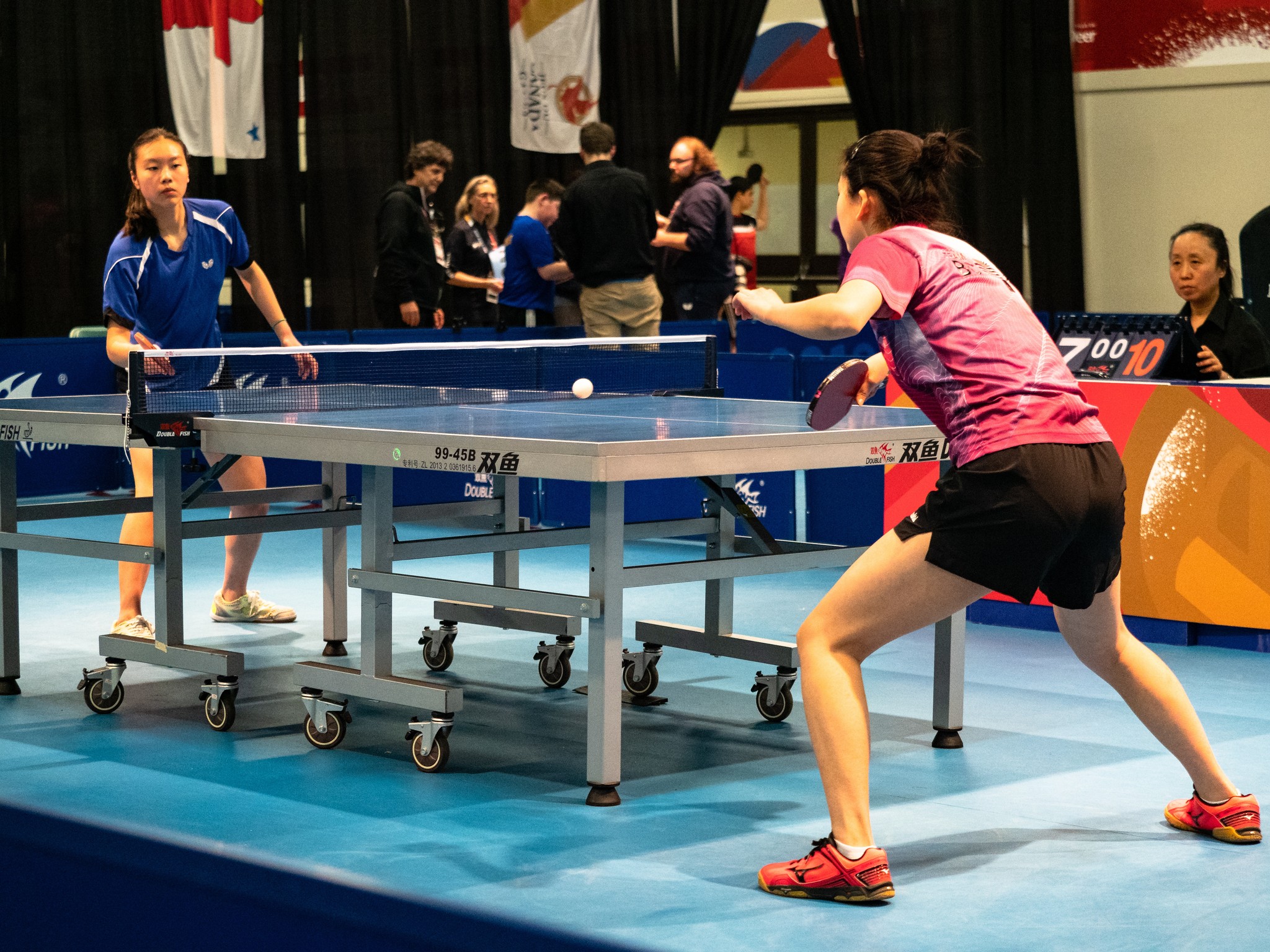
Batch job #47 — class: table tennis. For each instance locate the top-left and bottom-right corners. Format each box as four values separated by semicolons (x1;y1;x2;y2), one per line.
572;378;594;399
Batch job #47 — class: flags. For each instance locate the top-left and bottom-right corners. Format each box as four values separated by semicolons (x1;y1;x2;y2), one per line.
161;1;267;159
508;0;600;155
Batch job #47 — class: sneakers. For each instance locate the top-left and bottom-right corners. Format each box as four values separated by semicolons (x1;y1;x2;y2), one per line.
210;587;296;622
758;831;895;900
111;615;155;640
1164;781;1262;844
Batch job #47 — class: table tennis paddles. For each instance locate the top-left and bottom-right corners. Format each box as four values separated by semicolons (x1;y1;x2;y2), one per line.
804;357;878;433
134;331;179;377
746;163;770;185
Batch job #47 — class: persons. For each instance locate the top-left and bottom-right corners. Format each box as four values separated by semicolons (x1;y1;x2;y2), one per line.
724;175;769;289
731;125;1261;902
497;181;573;327
104;126;318;643
372;141;453;329
444;175;505;328
649;137;736;319
1168;224;1270;379
557;122;664;351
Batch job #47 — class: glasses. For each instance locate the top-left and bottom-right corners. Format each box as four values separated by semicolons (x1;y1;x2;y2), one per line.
669;157;698;165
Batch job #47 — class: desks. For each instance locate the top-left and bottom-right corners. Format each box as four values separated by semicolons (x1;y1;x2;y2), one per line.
884;378;1270;654
0;384;964;807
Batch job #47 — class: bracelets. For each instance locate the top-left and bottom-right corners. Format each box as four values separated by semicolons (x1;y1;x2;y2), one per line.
272;319;286;329
1227;373;1230;379
